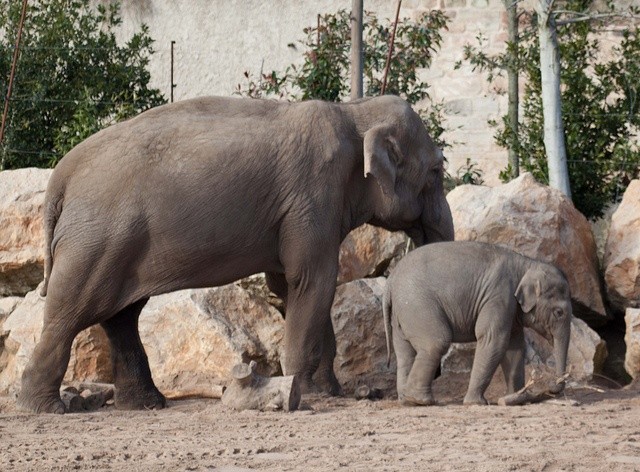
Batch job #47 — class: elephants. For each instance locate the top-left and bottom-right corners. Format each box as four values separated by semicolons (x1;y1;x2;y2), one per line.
14;93;456;415
381;240;575;406
